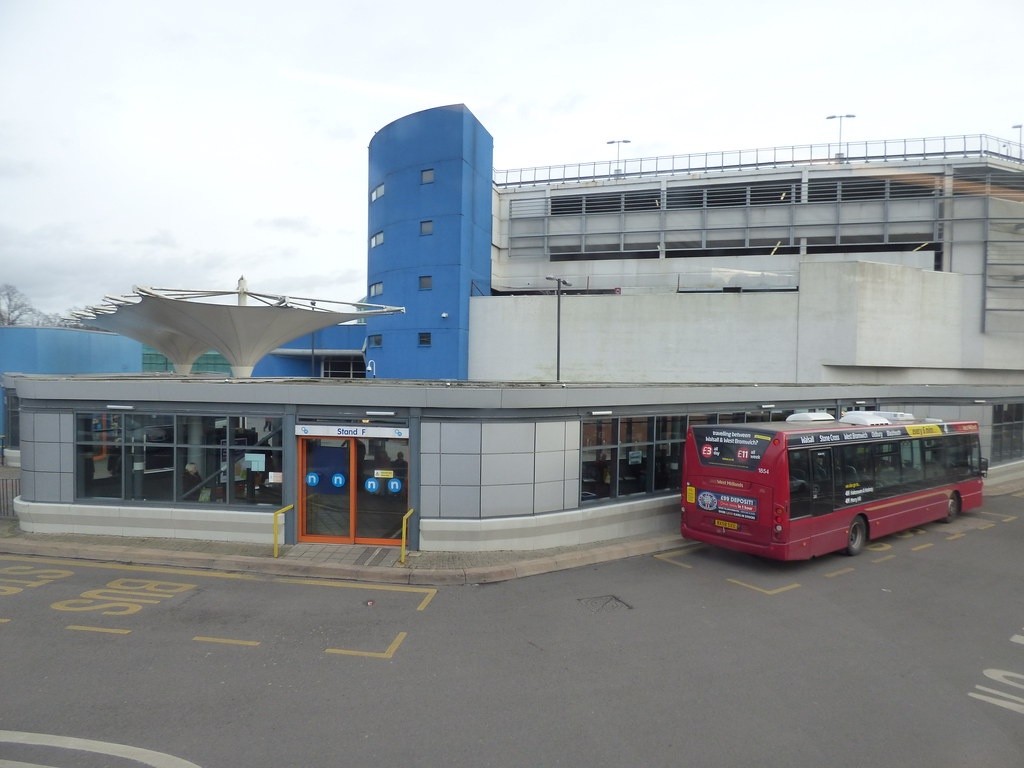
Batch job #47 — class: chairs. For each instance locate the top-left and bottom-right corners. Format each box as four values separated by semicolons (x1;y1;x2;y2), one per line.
793;465;860;485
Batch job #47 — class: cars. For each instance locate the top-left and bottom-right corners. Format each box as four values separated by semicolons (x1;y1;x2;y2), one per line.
78;413;169;450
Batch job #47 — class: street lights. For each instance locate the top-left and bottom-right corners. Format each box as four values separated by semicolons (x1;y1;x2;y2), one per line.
826;115;856;163
1013;125;1021;164
548;276;573;383
606;140;631;178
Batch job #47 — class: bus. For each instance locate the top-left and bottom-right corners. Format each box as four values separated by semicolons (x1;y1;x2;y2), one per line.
583;417;676;446
679;409;990;562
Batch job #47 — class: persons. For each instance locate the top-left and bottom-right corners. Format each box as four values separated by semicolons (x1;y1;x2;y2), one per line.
596;453;610;496
263;417;272;431
108;438;134;475
258;440;272;482
343;440;366;465
394;452;408;481
183;463;202;501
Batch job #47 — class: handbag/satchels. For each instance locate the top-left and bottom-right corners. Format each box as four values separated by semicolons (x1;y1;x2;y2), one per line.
198;485;212;504
